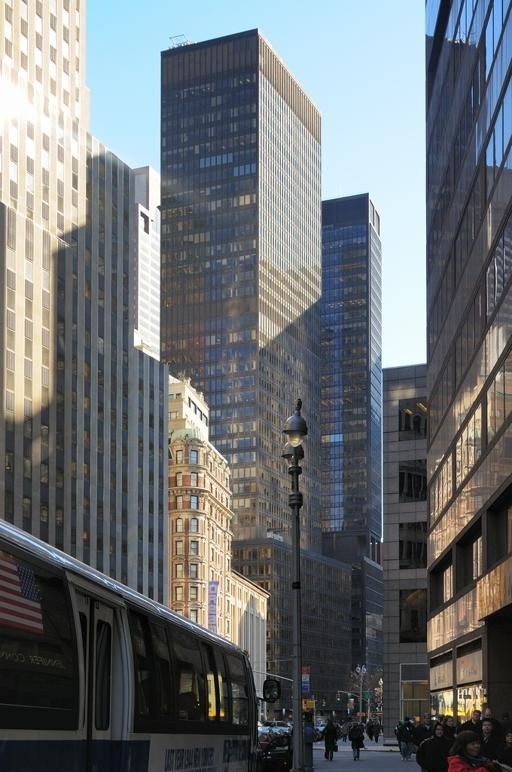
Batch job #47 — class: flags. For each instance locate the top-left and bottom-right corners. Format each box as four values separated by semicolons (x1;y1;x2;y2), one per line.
0;550;44;635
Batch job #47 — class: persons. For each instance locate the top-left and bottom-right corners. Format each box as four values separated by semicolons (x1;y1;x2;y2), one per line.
320;707;511;772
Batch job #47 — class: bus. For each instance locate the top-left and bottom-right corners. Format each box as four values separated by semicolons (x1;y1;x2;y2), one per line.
0;518;283;770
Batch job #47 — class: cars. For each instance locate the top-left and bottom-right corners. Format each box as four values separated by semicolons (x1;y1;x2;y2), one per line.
256;718;329;772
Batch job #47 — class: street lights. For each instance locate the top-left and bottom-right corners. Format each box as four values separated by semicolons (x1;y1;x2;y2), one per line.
378;678;384;735
354;664;368;723
281;395;317;771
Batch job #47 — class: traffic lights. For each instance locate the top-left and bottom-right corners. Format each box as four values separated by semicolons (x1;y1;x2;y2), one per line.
336;694;341;705
